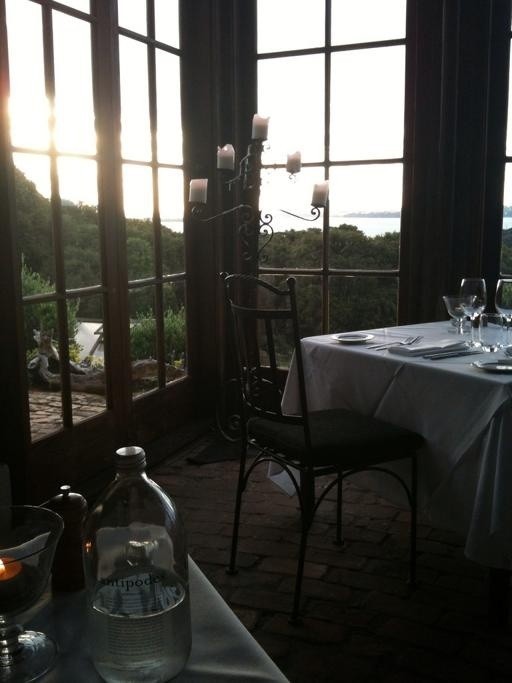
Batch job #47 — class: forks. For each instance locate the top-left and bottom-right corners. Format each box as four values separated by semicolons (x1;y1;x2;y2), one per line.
366;333;428;352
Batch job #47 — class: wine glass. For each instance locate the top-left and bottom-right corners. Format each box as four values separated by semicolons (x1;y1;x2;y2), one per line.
1;502;65;682
442;293;477;338
458;277;487;349
494;279;512;355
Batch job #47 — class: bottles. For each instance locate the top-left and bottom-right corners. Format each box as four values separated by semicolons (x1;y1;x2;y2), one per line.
42;484;97;564
81;444;191;682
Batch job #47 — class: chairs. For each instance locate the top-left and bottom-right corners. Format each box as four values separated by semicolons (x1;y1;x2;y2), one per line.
216;269;427;624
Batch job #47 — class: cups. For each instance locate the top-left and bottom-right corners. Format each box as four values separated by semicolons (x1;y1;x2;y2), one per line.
478;311;503;351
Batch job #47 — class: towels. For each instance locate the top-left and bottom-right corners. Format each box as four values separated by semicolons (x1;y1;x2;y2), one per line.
388;337;470;356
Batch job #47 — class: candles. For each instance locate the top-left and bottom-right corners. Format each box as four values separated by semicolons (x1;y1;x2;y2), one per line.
311;180;329;206
286;151;300;171
217;144;234;170
251;114;269;139
189;178;208;205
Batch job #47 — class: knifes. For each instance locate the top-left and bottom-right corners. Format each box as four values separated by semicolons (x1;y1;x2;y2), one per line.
422;350;484;360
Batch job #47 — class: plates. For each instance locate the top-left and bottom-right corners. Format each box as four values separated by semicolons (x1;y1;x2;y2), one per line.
471;358;512;372
330;331;376;343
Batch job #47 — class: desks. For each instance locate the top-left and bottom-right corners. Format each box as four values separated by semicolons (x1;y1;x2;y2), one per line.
0;524;290;683
